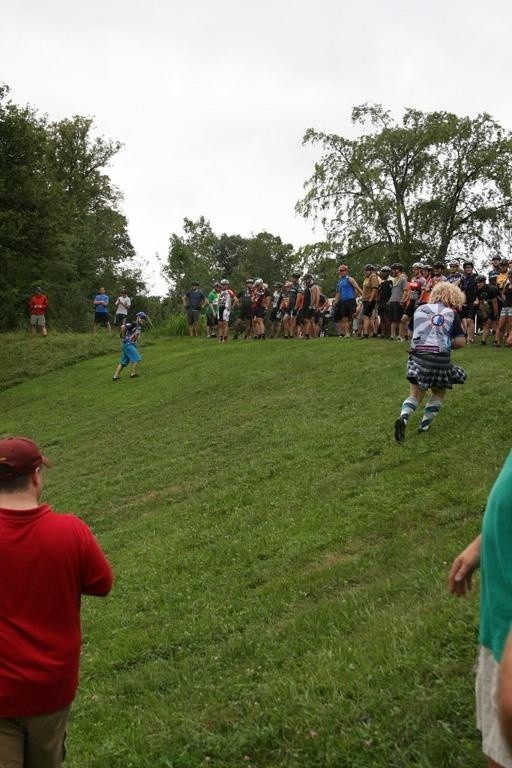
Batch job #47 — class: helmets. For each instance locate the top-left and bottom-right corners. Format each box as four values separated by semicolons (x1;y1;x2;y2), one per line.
336;264;348;275
136;312;146;318
272;272;312;292
492;255;508;265
212;279;229;287
246;277;264;287
364;260;486;284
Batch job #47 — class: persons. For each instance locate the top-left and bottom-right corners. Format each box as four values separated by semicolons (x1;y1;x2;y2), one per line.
181;280;207;337
113;289;132;338
29;287;49;337
112;311;146;381
395;282;467;446
0;433;113;768
92;286;112;336
449;448;512;768
205;255;511;349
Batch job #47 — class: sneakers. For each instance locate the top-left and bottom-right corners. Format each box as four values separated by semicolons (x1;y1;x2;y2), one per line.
130;374;138;378
395;418;406;444
112;377;121;381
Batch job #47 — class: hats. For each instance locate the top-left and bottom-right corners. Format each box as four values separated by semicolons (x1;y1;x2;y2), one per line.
0;436;49;480
